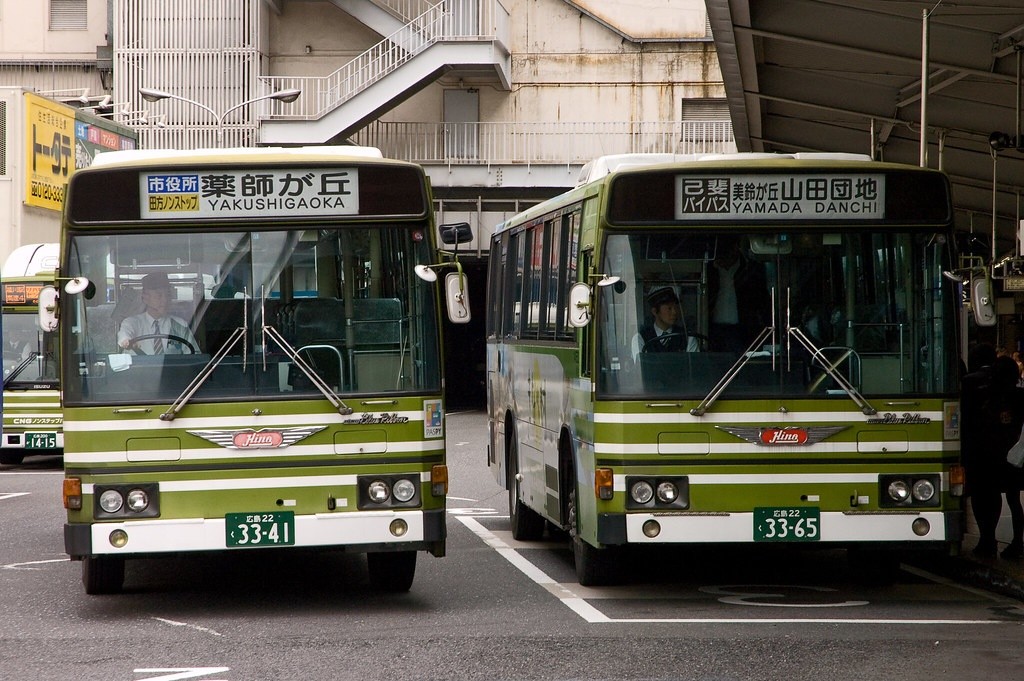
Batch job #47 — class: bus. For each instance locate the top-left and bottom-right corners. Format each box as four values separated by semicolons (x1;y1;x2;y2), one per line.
487;151;997;587
2;242;261;465
54;146;473;596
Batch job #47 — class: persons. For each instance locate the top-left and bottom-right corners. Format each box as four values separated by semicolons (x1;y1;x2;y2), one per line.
631;287;700;364
967;342;1024;560
118;271;202;356
3;329;32;361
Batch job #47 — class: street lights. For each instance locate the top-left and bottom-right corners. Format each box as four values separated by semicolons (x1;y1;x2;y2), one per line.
136;88;305;299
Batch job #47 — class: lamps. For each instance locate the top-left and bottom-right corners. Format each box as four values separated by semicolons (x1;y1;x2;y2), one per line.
458;79;464;88
305;46;310;53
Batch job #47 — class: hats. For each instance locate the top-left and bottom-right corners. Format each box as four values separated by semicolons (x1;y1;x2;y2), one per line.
141;273;172;290
647;287;679;306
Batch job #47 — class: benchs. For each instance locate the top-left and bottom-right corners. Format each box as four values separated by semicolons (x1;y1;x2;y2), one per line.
94;292;404;390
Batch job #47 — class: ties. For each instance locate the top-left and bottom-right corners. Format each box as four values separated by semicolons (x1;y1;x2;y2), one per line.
154;320;164;355
12;343;16;349
661;332;671;352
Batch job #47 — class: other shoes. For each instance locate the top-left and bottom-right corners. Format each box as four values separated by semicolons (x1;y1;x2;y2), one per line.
972;539;998;559
1000;543;1024;562
